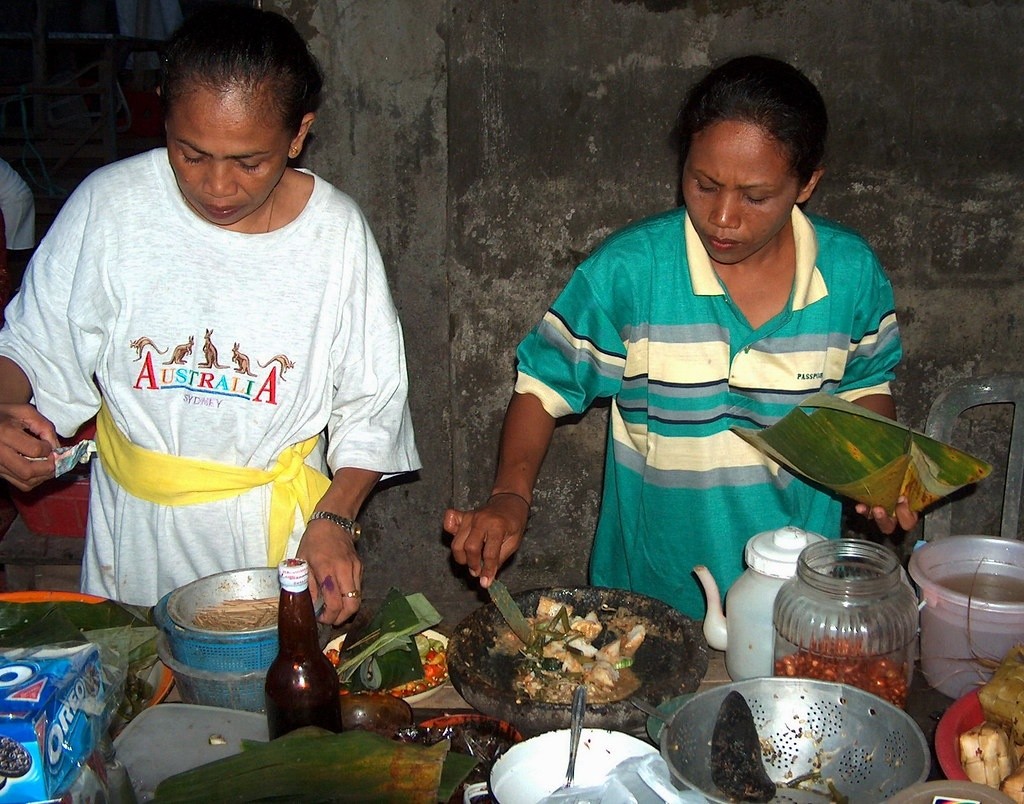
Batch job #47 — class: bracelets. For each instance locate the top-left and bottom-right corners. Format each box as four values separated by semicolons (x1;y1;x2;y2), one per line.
487;492;532;519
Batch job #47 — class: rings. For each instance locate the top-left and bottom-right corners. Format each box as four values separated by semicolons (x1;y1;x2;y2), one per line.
343;590;360;599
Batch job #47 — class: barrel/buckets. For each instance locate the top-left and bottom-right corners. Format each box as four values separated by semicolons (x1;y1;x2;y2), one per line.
909;534;1024;698
886;779;1017;804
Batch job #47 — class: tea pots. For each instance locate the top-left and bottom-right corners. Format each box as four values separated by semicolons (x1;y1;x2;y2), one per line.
694;525;835;680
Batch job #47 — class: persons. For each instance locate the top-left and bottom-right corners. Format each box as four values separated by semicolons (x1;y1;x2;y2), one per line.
0;156;33;329
0;7;423;627
445;56;917;622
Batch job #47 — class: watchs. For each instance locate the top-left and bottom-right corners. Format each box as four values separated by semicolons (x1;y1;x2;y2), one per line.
308;511;360;541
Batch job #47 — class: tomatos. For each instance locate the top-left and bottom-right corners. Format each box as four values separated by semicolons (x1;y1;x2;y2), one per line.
327;642;448;698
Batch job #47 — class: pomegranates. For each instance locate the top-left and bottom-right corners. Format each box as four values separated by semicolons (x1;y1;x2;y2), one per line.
775;637;910;711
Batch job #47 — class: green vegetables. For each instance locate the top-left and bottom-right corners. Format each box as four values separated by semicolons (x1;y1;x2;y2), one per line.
519;606;595;702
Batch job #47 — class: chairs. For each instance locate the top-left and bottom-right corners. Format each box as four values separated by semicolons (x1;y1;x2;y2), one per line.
922;372;1024;541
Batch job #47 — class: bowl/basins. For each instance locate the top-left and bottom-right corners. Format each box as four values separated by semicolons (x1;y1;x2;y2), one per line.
489;728;670;804
445;587;710;733
646;693;696;742
936;685;986;782
339;691;412;740
166;568;325;634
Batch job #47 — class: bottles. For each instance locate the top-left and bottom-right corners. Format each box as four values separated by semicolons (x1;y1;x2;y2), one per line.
265;558;343;742
772;538;918;712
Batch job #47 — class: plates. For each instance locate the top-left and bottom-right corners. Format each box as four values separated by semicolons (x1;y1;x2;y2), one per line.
400;714;523;744
323;626;451;704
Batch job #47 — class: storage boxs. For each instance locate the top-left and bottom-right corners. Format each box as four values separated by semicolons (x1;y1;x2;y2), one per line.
0;640;120;804
